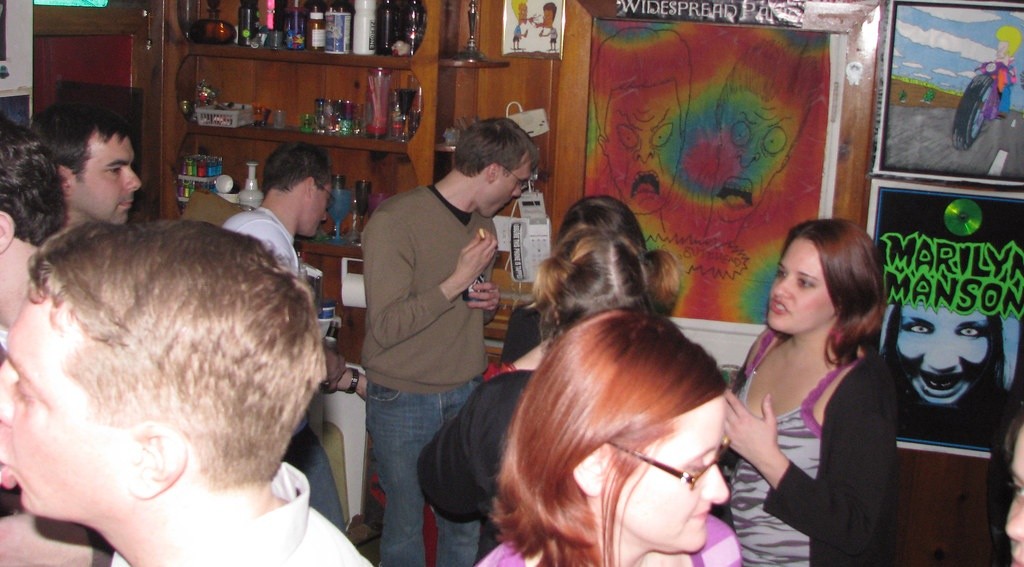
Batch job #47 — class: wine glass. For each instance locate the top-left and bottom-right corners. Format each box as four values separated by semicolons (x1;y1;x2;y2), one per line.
320;174;394;247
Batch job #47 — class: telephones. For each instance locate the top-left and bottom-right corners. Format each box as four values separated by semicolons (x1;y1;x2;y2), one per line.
508;216;551;283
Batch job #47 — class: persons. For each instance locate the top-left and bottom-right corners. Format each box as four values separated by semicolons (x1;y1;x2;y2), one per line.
225;142;368;535
361;116;538;567
0;221;374;567
418;196;743;567
721;218;898;567
0;99;140;567
991;404;1024;567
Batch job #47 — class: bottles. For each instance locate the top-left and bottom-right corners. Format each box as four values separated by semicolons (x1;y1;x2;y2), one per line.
187;2;427;57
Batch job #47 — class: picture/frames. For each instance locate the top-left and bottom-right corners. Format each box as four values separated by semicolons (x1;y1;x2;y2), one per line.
502;1;565;61
865;177;1024;459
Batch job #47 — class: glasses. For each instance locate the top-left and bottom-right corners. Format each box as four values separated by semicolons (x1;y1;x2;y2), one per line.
301;173;337;211
479;160;534;193
607;436;732;492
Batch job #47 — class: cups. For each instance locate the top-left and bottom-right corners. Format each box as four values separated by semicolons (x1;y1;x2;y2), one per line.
258;65;420;146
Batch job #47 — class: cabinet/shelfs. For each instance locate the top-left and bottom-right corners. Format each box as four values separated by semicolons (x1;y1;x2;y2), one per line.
436;59;510;152
157;0;437;372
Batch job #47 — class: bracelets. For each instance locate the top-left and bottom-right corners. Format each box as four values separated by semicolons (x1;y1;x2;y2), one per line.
347;368;359;394
